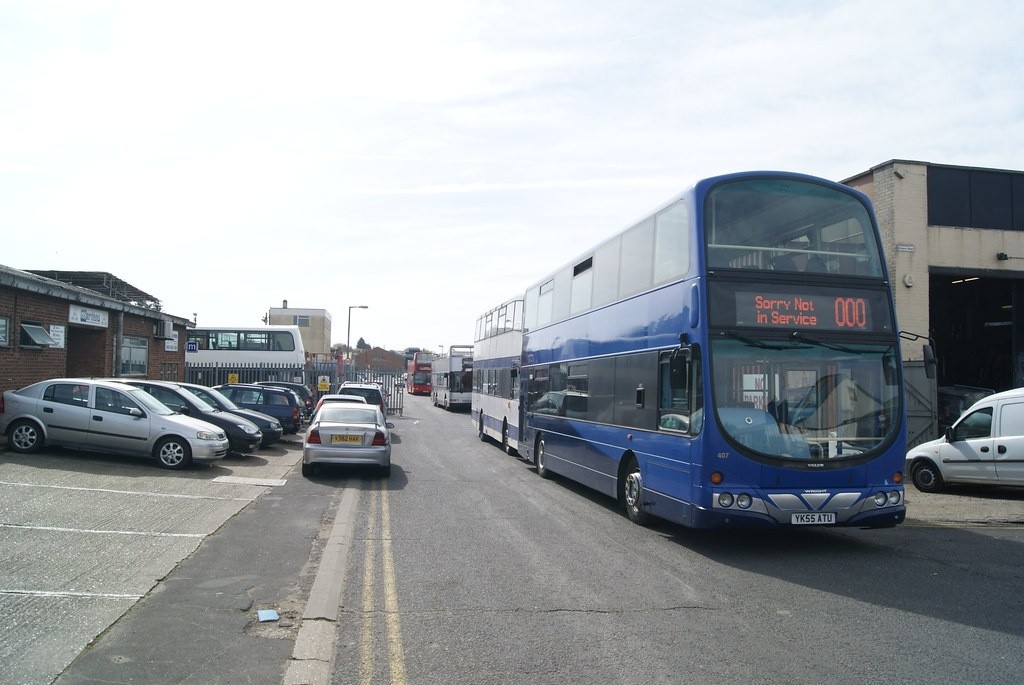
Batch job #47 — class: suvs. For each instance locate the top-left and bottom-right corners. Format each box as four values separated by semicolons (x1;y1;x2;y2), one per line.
338;381;388;418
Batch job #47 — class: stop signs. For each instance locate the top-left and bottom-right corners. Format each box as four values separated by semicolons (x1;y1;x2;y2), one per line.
344;359;351;366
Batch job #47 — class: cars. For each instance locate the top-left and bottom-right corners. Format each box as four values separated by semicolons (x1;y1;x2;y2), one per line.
301;403;394;476
394;378;406;389
75;379;263;457
0;378;230;470
308;394;368;426
164;380;285;447
174;381;317;435
401;373;408;379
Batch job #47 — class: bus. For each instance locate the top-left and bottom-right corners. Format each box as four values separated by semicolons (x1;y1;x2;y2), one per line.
517;169;938;529
117;326;306;387
469;296;527;452
430;344;474;411
406;353;439;396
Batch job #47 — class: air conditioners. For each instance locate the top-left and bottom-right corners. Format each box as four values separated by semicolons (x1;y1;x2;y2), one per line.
157;321;173;338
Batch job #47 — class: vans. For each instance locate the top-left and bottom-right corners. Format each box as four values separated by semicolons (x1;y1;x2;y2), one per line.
905;384;1024;493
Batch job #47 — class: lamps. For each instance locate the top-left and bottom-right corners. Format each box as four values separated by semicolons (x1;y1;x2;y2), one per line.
894;168;906;179
998;252;1007;260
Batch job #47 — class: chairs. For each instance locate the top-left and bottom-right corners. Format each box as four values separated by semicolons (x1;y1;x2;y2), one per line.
717;398;762;409
774;256;798;270
806;256;827;272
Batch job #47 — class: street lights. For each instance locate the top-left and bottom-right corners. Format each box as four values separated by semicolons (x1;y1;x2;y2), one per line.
439;345;444;361
347;305;369;360
